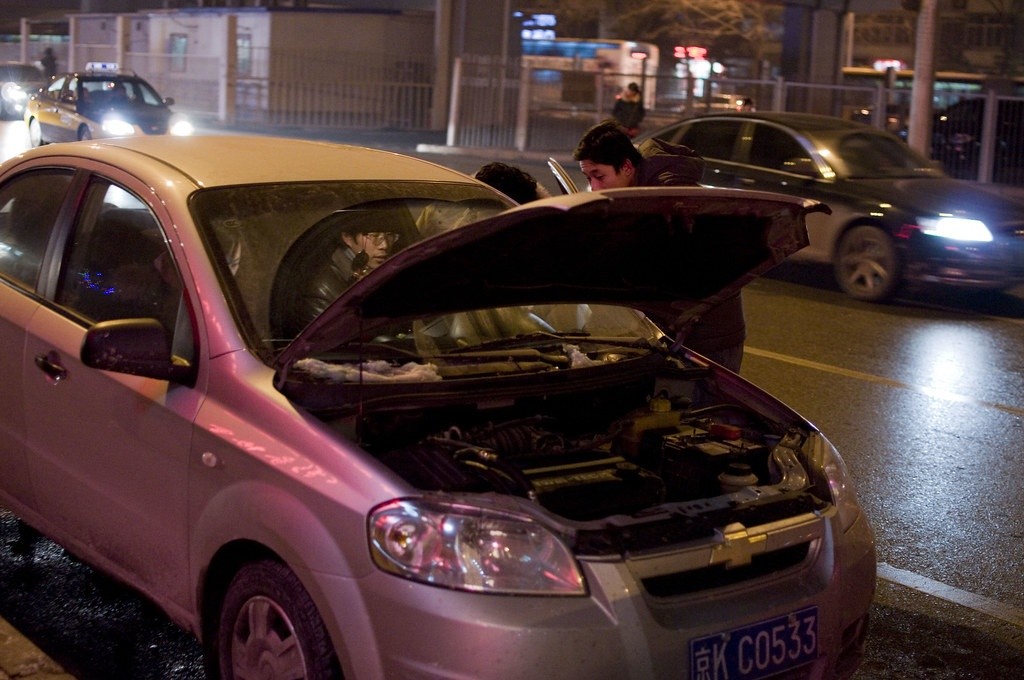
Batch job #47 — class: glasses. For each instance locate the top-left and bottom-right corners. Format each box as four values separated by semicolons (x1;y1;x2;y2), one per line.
365;233;400;247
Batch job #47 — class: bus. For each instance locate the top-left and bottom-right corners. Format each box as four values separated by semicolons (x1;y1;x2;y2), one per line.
841;67;1024;135
521;39;660;128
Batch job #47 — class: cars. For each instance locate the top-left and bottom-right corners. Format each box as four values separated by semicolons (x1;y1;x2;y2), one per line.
1;136;877;680
897;98;1024;163
692;94;734;109
586;112;1024;302
0;61;47;121
23;62;196;149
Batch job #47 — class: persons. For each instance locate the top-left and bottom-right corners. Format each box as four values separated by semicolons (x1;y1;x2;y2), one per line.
133;222;240;367
290;202;403;341
416;161;591;341
41;48;58;82
572;119;746;376
612;82;645;139
